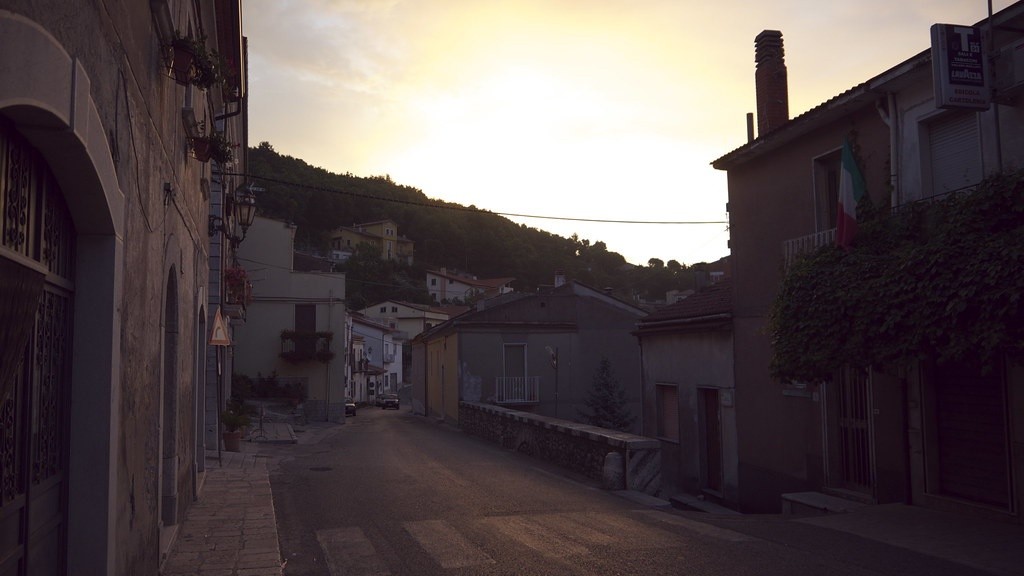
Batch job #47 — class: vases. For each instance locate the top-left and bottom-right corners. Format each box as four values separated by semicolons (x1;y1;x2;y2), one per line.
194;141;209;155
198;152;210;162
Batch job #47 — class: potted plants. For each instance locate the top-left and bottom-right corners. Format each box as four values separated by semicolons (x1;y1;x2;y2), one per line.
304;331;336;351
238;374;253;397
256;368;280;398
282;381;309;407
280;326;299;352
176;27;238;93
221;406;249;452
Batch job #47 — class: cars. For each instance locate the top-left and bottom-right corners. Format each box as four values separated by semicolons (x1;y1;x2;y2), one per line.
382;394;400;410
344;397;358;417
376;393;385;404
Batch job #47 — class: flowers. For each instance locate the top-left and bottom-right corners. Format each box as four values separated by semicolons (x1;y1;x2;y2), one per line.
186;119;241;167
225;266;256;292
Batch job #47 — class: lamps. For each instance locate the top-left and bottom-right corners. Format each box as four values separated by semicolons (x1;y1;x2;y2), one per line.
208;193;258;253
